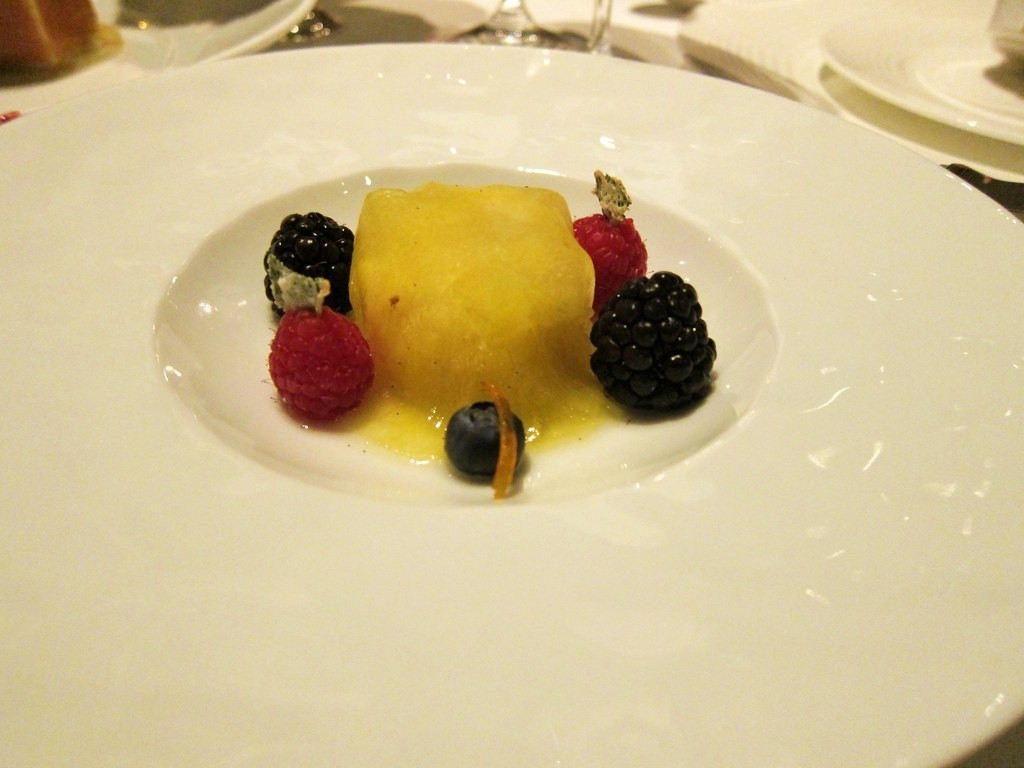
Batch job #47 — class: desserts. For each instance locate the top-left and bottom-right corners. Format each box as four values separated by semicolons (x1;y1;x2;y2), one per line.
349;184;596;422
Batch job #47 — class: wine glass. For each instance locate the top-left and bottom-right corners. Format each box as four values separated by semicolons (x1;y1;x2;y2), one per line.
435;0;585;49
579;0;627;58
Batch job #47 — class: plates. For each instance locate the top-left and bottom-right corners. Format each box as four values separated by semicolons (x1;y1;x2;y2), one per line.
816;0;1024;146
0;40;1024;768
1;0;318;107
